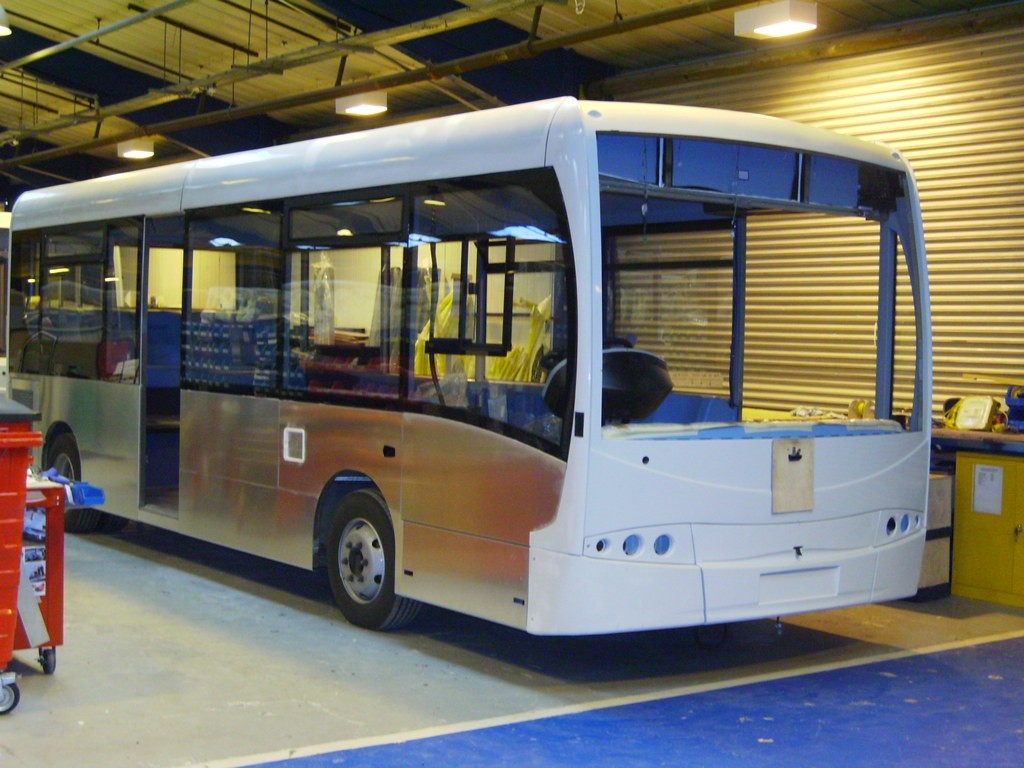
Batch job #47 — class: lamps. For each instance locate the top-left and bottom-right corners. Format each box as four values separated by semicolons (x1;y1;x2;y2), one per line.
734;0;817;40
118;140;155;159
336;92;388;115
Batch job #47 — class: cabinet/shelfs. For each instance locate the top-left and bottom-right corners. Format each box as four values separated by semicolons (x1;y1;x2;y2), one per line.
951;453;1024;609
12;478;66;673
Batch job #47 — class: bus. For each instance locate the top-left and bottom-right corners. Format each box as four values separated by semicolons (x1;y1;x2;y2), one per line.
4;95;935;637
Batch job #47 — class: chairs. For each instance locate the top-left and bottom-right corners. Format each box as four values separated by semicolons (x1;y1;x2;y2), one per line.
44;307;181;385
